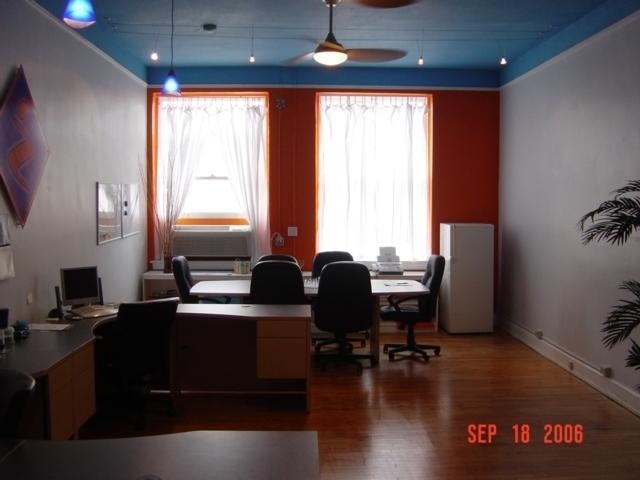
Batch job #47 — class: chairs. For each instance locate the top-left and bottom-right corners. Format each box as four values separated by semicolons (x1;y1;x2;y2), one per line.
101;297;181;431
172;253;446;376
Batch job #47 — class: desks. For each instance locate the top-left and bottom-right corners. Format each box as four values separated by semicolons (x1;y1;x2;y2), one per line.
0;302;311;441
141;270;437;333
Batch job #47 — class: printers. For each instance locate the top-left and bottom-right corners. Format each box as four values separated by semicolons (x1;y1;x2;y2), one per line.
375;256;403;274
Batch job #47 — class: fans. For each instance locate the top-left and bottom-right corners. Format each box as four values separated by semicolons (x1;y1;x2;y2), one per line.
280;0;407;67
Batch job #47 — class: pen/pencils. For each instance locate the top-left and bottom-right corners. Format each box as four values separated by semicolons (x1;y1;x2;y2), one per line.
398;283;407;284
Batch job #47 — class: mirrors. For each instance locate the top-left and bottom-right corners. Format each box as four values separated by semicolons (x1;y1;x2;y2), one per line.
96;181;122;245
122;179;141;239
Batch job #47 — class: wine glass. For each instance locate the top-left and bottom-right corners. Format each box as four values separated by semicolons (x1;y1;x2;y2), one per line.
298;260;304;272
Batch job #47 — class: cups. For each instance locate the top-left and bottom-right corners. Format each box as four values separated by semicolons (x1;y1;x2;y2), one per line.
303;277;319;289
232;261;250;274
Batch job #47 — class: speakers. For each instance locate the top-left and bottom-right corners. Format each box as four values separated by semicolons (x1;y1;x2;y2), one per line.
55;286;63;320
98;278;104;305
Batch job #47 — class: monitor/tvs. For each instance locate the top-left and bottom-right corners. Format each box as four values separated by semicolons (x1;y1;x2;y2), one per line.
60;265;101;312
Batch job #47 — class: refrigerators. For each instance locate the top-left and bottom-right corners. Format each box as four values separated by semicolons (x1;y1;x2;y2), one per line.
438;221;494;334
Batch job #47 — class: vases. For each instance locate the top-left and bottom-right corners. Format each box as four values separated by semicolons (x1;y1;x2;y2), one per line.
164;255;173;273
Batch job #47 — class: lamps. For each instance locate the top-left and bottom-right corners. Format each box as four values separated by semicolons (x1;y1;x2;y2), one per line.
500;40;507;64
61;0;97;30
313;51;348;68
164;0;180;92
417;28;425;65
151;30;161;60
250;24;256;63
